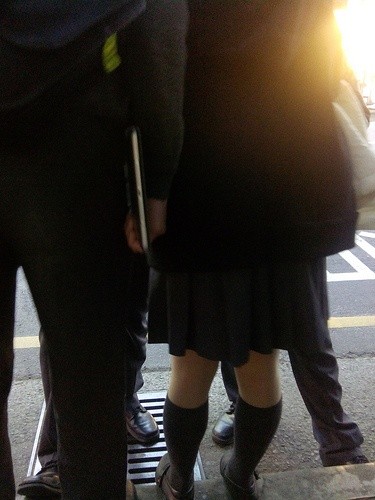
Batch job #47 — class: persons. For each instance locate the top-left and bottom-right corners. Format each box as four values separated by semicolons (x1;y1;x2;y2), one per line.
0;0;375;500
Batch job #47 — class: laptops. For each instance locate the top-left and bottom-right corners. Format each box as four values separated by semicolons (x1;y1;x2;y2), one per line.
126;126;153;252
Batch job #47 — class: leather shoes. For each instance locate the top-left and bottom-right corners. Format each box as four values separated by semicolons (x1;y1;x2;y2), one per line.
212;404;235;447
126;396;159;447
220;452;257;500
155;452;195;500
342;454;370;465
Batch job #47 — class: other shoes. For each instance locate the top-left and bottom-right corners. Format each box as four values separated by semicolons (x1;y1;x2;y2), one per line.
16;461;62;498
